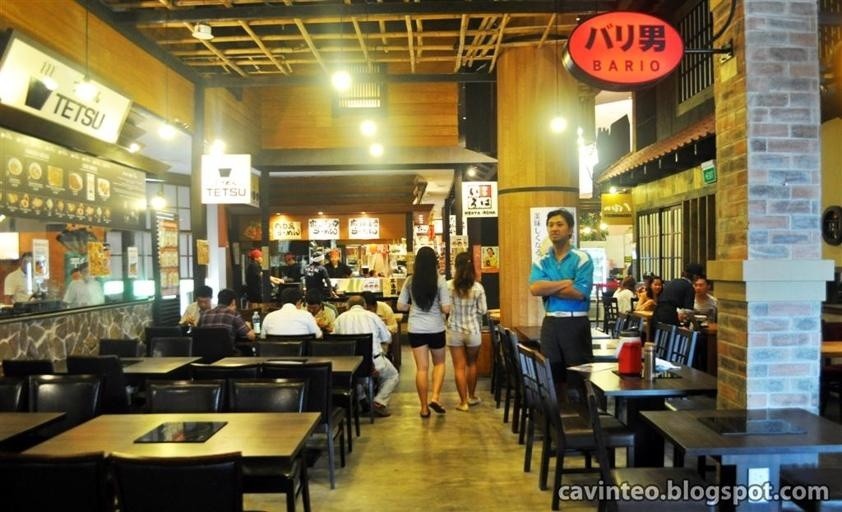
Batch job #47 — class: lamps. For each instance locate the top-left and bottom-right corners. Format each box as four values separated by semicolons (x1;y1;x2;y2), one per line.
190;19;213;42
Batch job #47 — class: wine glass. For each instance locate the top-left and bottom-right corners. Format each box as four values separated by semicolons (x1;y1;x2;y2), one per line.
186;311;196;332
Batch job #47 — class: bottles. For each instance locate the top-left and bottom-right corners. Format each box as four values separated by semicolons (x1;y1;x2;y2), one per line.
252;310;261;334
643;342;657;381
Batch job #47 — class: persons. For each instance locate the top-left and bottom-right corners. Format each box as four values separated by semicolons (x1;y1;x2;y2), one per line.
3;246;399;418
482;247;497;267
608;262;717;356
396;246;453;419
445;251;488;410
525;211;596;403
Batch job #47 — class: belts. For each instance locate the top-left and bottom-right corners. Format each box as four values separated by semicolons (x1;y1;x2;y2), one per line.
544;311;588;317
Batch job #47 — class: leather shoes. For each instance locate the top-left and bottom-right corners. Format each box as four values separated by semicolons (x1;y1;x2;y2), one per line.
468;397;481;405
456;403;468;412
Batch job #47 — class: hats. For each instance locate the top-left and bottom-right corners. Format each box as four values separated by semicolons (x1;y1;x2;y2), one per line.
685;264;704;275
325;250;340;260
250;250;262;259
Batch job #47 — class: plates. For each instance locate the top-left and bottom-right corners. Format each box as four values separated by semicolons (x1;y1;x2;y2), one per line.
69;173;83;191
98;178;111;197
30;162;43;180
8;158;23;175
48;166;64;186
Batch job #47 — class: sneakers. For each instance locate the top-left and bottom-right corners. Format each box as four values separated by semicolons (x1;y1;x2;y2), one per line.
370;402;391;417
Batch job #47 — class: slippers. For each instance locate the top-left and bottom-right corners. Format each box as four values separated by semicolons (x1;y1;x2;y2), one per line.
430;401;445;413
421;408;431;417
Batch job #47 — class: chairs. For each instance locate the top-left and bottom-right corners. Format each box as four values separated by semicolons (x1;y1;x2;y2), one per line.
483;282;842;512
0;306;405;512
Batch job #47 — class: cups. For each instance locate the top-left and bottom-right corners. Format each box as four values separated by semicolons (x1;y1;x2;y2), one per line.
25;75;59;110
